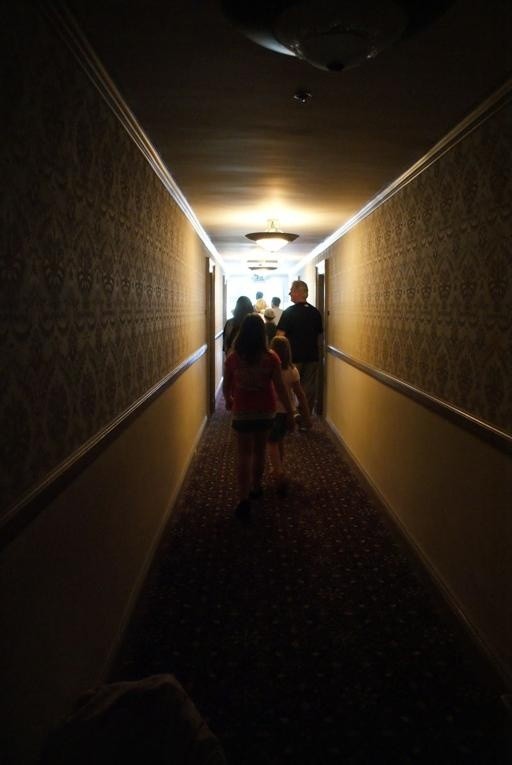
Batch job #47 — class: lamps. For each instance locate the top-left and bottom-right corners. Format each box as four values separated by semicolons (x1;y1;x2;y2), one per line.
243;218;299;273
220;0;455;74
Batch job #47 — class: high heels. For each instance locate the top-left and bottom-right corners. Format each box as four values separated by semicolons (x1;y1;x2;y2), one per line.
250;482;268;505
237;501;252;535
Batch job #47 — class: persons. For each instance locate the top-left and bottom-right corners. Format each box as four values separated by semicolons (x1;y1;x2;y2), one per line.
221;280;326;504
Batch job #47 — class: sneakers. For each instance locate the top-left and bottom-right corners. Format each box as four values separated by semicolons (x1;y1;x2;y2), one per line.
298;423;309;431
